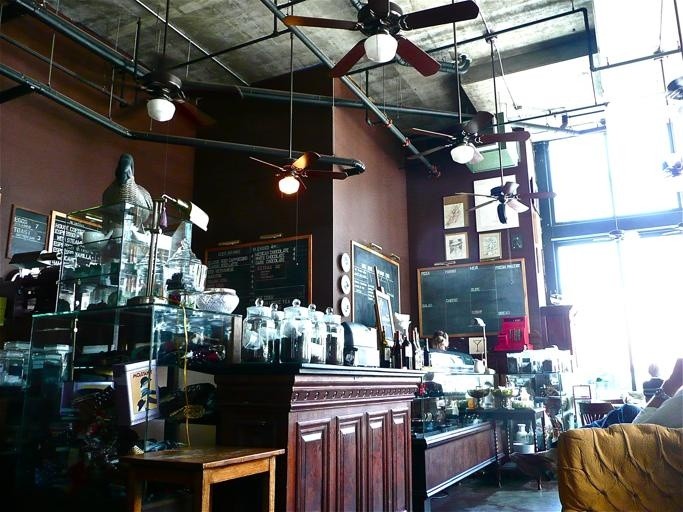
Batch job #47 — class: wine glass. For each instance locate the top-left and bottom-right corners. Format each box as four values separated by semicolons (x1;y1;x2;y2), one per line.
473;339;483;353
467;386;520;411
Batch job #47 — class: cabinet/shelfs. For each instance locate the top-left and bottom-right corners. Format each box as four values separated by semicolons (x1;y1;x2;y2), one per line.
220;361;425;511
2;202;244;512
412;314;566;512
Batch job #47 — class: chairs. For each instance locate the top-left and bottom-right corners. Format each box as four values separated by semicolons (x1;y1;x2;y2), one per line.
557;384;683;512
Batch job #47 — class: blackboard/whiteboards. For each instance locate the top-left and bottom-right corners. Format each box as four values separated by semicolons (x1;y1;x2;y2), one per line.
417;257;532;338
7;204;109;269
352;240;401;330
206;235;312;313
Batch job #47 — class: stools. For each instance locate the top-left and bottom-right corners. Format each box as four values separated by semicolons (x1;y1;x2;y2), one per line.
122;449;286;512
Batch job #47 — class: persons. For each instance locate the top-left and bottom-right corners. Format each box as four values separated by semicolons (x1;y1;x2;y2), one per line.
532;359;564;393
448;239;462;258
641;362;665;404
629;357;682;430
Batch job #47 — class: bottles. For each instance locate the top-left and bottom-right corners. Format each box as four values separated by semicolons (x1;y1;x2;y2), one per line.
242;298;345;366
6;349;23;385
164;238;239;315
516;421;534;444
379;328;430;370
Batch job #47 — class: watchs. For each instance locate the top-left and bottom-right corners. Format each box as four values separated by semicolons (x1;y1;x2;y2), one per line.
653;388;671;401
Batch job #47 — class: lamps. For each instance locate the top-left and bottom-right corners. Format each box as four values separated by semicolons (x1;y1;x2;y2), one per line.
146;92;176;123
451;138;475;165
364;25;398;64
278;171;300;195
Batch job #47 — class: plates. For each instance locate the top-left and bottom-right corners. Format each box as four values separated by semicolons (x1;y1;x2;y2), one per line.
340;253;351;317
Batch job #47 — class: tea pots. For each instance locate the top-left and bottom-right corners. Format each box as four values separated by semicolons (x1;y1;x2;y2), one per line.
471;358;486;373
81;228;114;250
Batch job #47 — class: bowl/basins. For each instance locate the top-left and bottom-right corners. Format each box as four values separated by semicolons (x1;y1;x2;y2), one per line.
512;443;535;454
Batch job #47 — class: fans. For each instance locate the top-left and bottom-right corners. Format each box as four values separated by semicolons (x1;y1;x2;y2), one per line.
113;0;244;126
405;0;530;160
282;0;479;78
454;32;556;224
249;1;348;191
